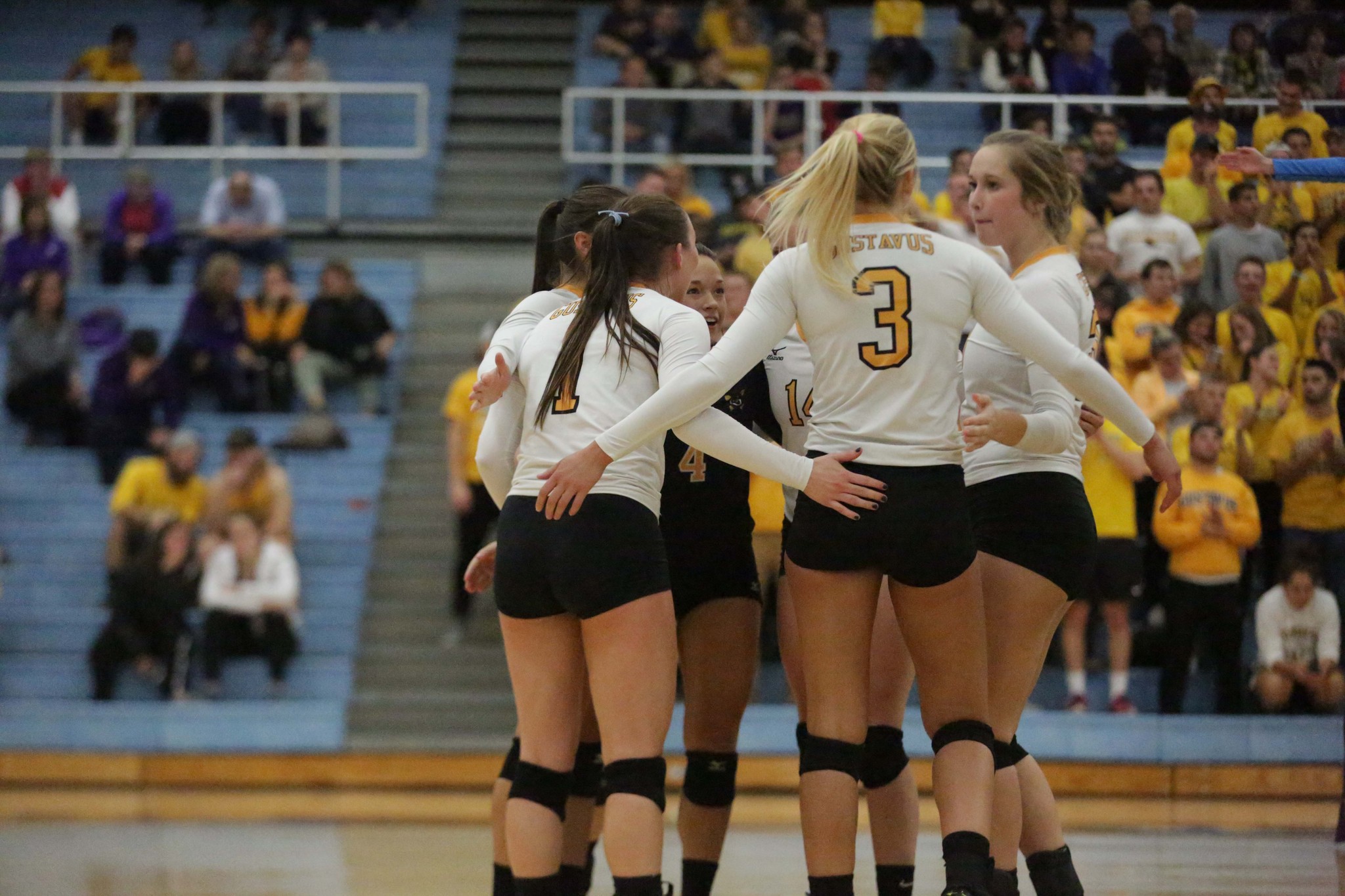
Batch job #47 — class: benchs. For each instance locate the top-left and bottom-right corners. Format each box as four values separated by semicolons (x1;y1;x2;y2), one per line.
0;0;461;754
564;0;1345;765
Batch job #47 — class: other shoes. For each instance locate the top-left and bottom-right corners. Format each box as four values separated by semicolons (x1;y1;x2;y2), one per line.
1108;697;1137;714
1067;694;1089;711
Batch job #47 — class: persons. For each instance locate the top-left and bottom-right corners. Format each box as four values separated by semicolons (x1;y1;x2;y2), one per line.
535;112;1183;896
2;0;1345;896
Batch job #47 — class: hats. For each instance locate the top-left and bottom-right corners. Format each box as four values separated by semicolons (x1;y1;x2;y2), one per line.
130;329;157;356
1189;76;1228;104
1322;126;1345;145
1194;135;1220;153
1264;141;1293;160
226;427;256;446
